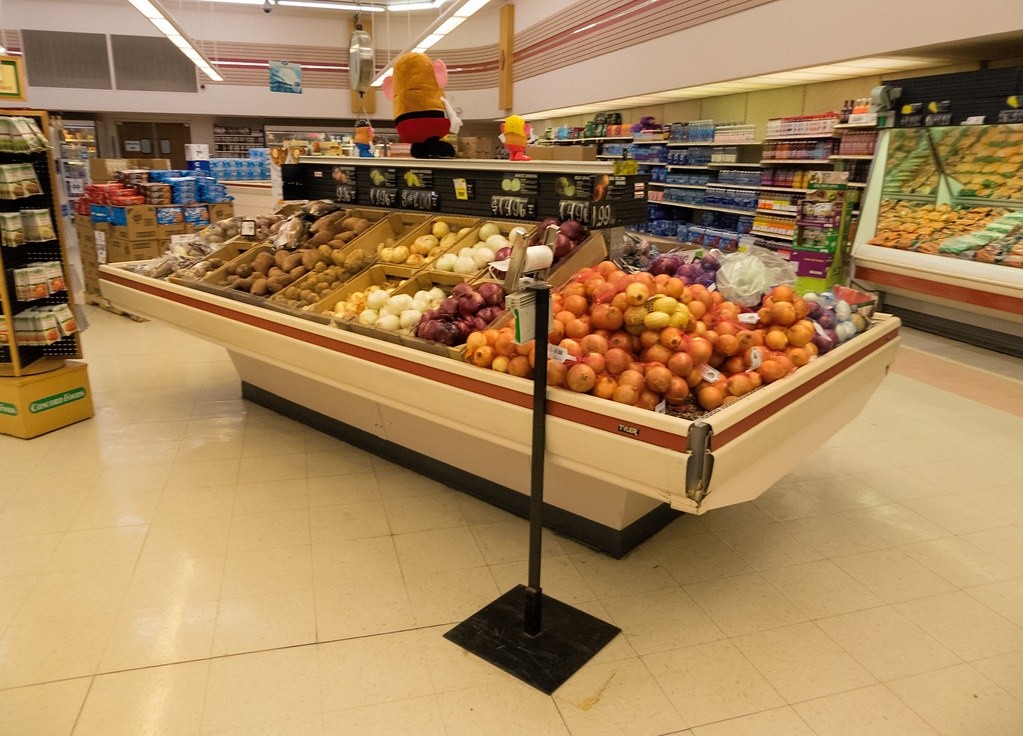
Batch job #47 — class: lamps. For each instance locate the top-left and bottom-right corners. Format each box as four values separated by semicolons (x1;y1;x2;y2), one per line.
127;0;492;86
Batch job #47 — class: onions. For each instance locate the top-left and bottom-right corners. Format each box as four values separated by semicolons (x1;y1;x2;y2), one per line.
416;216;839;415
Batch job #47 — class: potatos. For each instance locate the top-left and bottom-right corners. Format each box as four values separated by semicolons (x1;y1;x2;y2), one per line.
149;199;396;311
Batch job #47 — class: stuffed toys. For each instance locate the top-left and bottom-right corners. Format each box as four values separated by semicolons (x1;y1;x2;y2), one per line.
382;52;462;159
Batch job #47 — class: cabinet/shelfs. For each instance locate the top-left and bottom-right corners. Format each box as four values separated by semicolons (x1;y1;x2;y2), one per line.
537;124;878;242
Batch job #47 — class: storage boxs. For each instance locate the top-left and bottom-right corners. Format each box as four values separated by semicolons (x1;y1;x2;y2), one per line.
167;203;607;362
525;144;552;160
552;143;597;161
76;202;235;311
88;158;171;184
